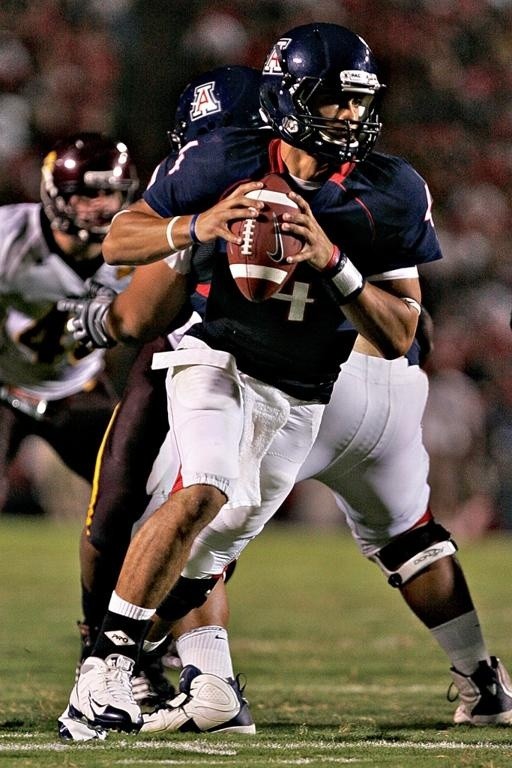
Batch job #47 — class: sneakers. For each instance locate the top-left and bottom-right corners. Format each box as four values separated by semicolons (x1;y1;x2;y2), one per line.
128;658;161;705
68;652;143;730
450;654;512;726
139;664;258;737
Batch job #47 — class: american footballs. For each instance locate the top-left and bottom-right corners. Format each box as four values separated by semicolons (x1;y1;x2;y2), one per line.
227;174;305;303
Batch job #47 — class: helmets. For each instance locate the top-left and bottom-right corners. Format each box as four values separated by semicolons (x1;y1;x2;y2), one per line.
258;22;384;164
40;132;140;244
167;66;267;164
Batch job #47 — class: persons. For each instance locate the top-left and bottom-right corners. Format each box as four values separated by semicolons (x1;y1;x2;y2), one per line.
0;129;141;488
0;1;512;539
56;60;511;734
57;19;443;744
77;334;172;666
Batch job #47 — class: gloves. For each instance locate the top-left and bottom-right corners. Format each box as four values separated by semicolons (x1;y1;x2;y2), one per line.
58;278;118;360
